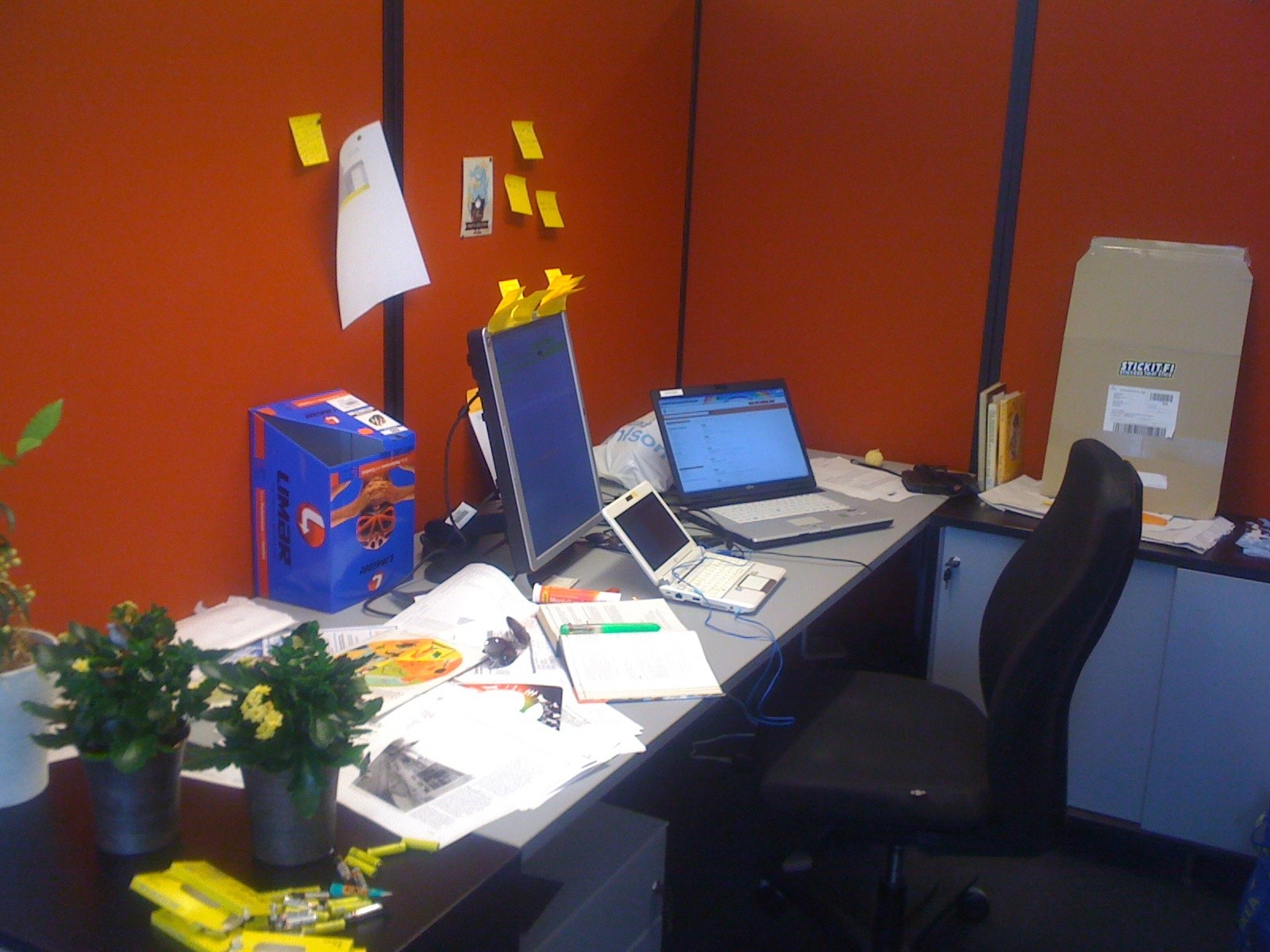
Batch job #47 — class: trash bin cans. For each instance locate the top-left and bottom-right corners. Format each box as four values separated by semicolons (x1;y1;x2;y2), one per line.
1238;808;1269;952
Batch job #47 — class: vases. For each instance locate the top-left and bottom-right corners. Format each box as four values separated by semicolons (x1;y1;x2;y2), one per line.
241;765;340;866
80;738;187;855
0;628;61;809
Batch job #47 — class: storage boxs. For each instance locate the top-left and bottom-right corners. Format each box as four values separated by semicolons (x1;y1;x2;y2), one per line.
1034;235;1254;520
247;389;416;614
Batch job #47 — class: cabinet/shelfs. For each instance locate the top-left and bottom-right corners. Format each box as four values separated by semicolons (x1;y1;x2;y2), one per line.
925;498;1270;860
521;800;669;951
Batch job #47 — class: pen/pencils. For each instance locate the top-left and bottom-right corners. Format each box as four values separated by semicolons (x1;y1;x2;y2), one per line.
559;622;662;634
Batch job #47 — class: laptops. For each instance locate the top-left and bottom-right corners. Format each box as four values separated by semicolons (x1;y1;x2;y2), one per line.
601;480;786;612
650;378;893;548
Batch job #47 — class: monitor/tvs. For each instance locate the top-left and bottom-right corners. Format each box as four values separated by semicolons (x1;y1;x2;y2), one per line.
467;308;604;574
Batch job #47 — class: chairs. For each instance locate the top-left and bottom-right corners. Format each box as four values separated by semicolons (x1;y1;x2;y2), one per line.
756;438;1144;951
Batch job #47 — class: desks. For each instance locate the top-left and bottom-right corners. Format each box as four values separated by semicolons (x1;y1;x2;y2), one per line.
0;447;977;951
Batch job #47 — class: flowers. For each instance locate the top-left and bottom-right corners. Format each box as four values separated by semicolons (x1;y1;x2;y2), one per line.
0;399;383;822
